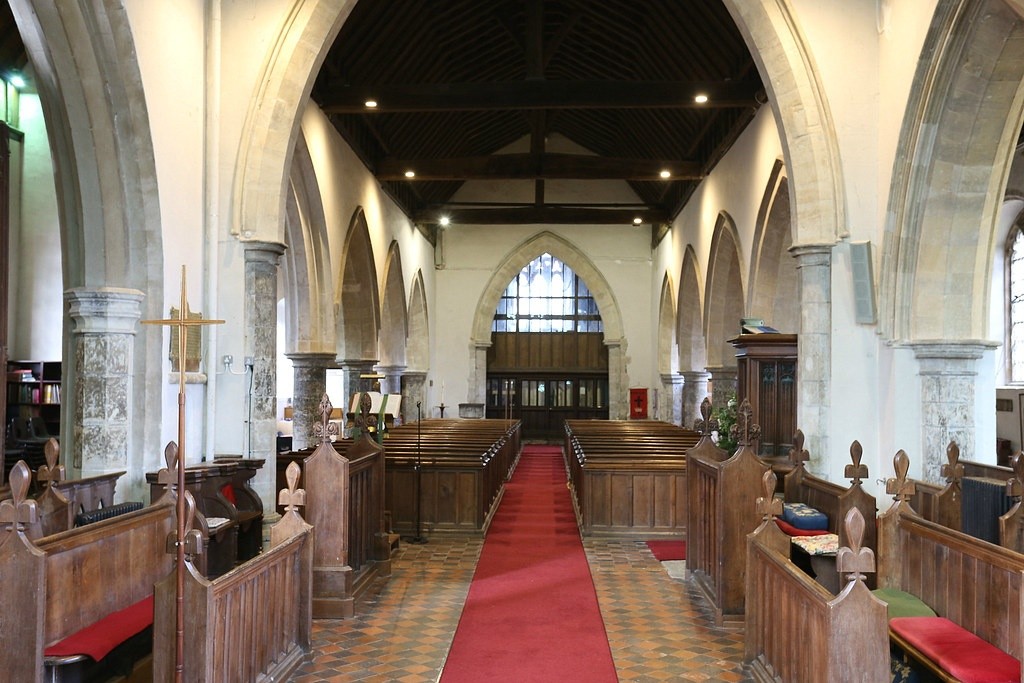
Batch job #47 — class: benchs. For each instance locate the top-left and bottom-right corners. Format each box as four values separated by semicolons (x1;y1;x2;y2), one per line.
563;419;708;541
43;595;154;682
871;588;1022;683
778;519;840;593
275;419;524;542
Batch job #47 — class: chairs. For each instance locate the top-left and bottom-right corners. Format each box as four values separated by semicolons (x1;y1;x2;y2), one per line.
4;416;63;482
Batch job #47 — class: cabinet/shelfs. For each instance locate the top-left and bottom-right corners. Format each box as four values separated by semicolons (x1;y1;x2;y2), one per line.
5;360;63;406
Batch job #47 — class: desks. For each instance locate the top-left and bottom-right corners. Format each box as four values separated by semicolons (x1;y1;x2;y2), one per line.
146;458;266;579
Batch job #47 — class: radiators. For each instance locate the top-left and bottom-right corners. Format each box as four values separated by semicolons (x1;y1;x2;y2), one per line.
76;502;143;526
961;477;1013;546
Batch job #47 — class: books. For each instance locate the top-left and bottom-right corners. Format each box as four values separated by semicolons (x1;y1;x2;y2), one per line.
44;384;60;403
8;370;36;382
9;383;39;416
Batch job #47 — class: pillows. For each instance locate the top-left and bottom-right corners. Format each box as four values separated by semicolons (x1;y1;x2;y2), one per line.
779;502;829;531
